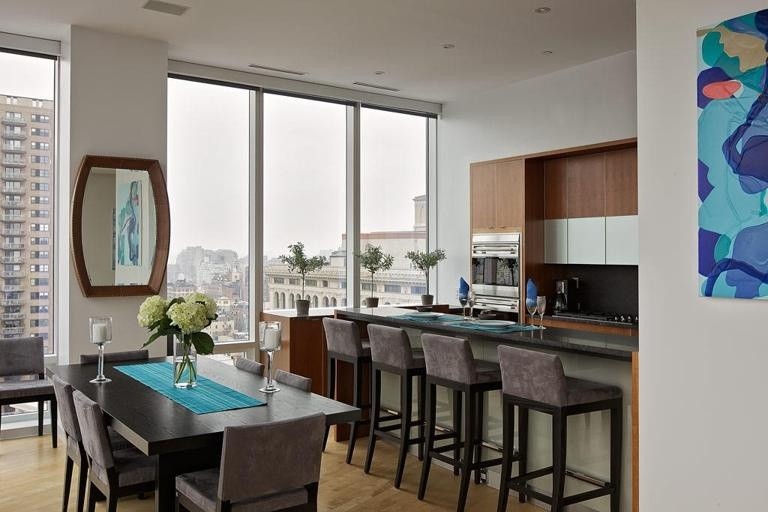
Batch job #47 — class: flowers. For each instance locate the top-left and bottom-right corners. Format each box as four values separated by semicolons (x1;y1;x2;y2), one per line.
133;293;220;382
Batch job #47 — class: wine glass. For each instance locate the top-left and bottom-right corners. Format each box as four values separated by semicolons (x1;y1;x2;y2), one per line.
87;315;112;384
525;296;548;330
458;290;477;321
259;322;281;393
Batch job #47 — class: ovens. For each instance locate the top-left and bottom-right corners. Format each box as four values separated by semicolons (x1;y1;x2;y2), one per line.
471;241;519;304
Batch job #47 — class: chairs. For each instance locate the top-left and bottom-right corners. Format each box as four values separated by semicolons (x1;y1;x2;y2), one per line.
72;390;159;512
274;369;313;394
171;408;327;512
47;377;146;512
78;347;148;365
0;333;61;450
236;356;262;375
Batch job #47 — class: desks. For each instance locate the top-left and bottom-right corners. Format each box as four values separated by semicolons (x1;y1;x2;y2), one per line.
43;354;364;512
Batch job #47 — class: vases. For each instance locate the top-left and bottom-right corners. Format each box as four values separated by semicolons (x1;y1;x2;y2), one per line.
172;333;198;389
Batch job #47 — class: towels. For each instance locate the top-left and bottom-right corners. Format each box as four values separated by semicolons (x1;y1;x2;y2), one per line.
458;277;470;304
525;279;534;310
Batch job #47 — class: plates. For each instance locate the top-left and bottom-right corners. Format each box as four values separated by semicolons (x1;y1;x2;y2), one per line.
404;312;445;319
468;320;517;328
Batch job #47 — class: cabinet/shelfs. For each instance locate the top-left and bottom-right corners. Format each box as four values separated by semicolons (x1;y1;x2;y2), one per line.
469;158;542;230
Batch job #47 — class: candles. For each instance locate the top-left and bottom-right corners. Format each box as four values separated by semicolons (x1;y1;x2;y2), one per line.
91;323;105;342
263;328;277;348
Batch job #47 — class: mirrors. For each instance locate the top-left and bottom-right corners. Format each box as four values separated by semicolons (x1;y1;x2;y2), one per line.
68;154;174;299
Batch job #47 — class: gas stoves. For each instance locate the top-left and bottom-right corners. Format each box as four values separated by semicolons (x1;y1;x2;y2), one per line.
551;312;637;327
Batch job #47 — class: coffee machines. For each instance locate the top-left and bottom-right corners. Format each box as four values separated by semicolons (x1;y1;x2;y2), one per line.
554;277;581;315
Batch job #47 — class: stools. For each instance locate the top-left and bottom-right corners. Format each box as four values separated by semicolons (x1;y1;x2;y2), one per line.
496;345;623;511
364;324;462;488
418;333;528;511
321;318;400;464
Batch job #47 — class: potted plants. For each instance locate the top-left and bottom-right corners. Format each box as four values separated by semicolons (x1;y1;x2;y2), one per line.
404;246;448;306
354;242;398;308
279;241;332;317
